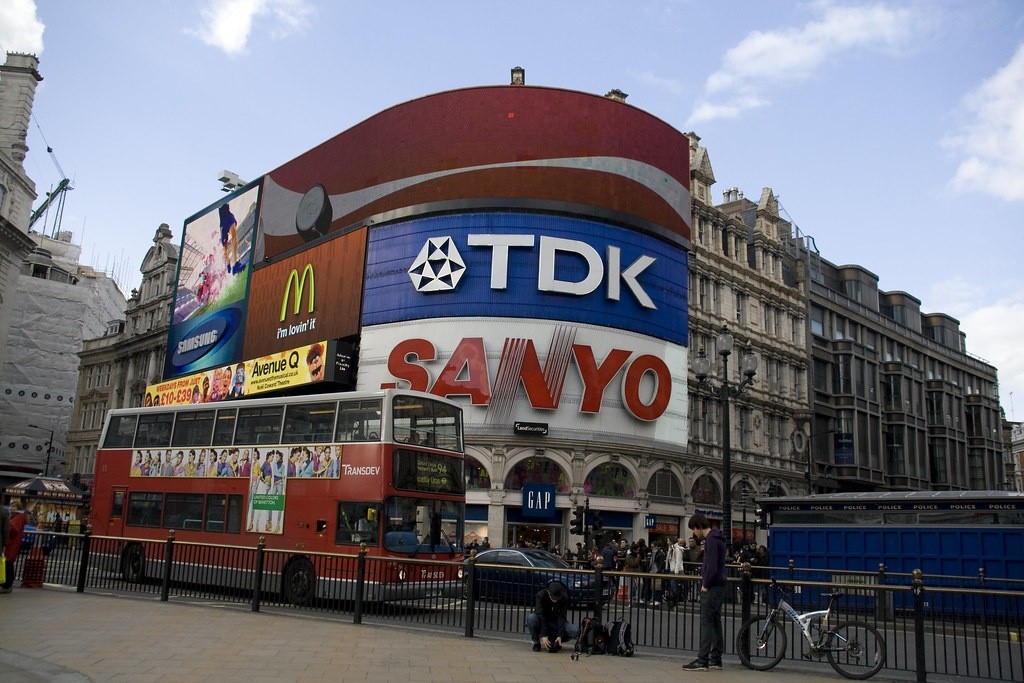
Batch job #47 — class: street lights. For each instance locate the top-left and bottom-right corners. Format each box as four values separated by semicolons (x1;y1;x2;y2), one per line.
690;325;761;605
28;423;55;477
806;426;845;496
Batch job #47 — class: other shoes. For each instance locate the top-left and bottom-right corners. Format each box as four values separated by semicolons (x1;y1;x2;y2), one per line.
650;600;661;606
639;599;644;603
0;586;13;594
532;642;541;652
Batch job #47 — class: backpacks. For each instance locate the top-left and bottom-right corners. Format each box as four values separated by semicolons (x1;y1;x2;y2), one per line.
604;616;634;657
574;616;606;655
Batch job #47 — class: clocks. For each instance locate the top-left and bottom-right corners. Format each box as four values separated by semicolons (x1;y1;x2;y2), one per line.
511;66;525;86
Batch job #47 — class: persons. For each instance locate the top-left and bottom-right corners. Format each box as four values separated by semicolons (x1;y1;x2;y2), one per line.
218;366;236;401
218;203;246;275
160;449;206;476
464;537;490;559
351;518;375;543
506;463;568;492
130;450;162;476
206;448;251;477
734;542;769;606
472;463;479;487
551;536;706;608
527;582;579;651
0;500;63;593
420;430;438;447
287;445;341;478
247;448;285;532
682;514;727;671
518;539;548;551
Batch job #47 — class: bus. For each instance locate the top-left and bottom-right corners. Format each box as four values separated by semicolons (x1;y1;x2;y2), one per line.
88;387;468;608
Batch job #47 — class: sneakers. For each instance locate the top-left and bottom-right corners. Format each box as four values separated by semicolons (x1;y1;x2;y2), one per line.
707;658;723;670
681;658;710;672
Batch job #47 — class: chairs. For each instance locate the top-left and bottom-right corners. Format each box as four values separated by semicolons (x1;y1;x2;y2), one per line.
118;426;243;445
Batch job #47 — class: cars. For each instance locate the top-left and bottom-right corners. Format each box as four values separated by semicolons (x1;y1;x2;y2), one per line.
4;506;58;557
464;547;615;612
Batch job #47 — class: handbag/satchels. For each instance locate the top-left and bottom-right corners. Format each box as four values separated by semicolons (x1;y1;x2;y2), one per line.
0;552;6;584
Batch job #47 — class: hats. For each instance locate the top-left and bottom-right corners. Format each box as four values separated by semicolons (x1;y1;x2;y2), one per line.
549;582;563;600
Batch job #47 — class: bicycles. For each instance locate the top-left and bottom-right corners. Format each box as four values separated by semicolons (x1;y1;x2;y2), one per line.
735;576;887;680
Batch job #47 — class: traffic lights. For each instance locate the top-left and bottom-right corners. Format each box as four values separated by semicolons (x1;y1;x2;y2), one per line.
755;507;768;531
590;509;603;531
570;506;583;536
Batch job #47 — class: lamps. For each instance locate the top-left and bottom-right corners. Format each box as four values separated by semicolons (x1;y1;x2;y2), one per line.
218;169;248;194
